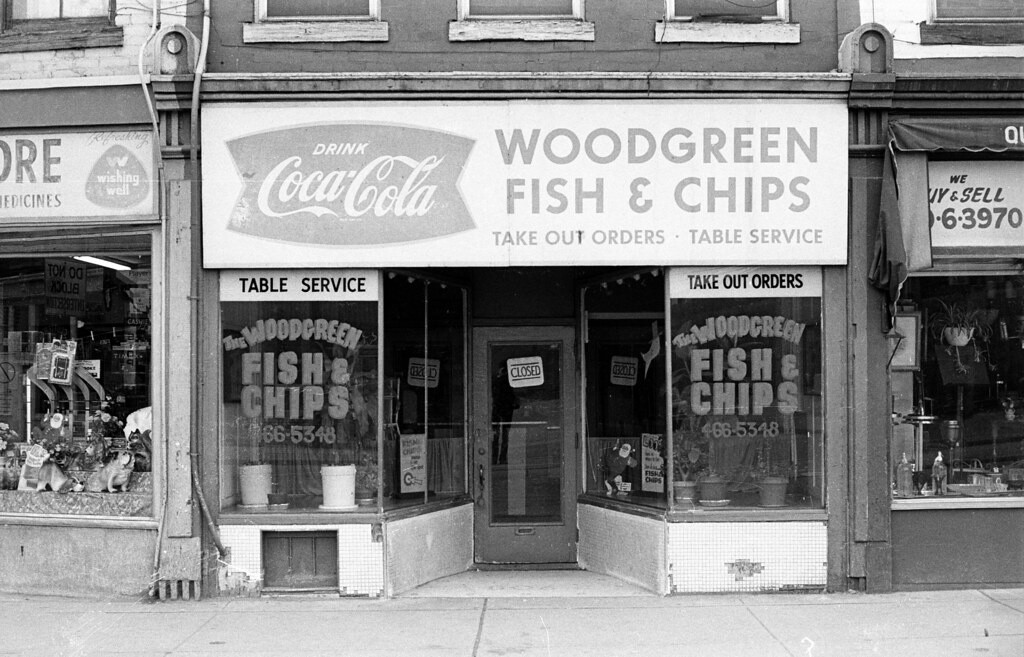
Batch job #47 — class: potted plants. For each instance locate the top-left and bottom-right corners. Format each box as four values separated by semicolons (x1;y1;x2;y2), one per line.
355;458;386;499
932;296;993;375
672;313;808;506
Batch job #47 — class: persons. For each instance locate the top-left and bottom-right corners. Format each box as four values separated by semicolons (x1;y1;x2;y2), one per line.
604;442;638;497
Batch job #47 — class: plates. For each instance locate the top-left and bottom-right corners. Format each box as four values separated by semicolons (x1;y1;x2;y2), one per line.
698;500;731;506
757;504;788;508
675;497;696;504
268;505;289;509
354;499;376;505
946;484;1024;497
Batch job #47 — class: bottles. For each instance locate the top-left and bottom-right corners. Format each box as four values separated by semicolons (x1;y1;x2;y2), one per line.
897;453;913;497
931;450;948;496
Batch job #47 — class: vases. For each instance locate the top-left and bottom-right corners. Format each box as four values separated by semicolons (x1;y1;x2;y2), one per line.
321;464;357;513
236;463;274;510
268;493;287;504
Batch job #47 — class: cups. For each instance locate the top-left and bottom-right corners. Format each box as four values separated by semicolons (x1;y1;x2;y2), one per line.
966;468;1024;490
757;475;790;504
701;475;727;500
267;492;289;503
675;480;698;497
356;489;374;498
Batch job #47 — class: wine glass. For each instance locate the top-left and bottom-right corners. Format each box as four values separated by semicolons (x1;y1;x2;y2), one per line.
913;471;927;496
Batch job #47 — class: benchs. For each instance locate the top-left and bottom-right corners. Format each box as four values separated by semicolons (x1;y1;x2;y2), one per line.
72;256;132;271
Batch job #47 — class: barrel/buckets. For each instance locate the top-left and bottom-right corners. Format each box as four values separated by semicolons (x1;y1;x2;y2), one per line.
319;464;356;505
240;465;273;505
